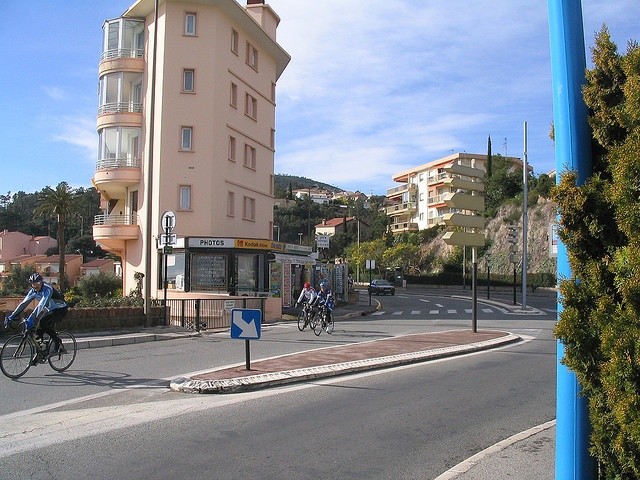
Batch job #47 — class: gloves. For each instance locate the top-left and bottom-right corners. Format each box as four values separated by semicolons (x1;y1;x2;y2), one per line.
7;315;16;320
27;316;33;328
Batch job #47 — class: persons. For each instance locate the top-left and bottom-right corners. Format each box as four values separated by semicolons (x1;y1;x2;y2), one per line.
295;282;317;327
313;282;335;326
4;273;68;366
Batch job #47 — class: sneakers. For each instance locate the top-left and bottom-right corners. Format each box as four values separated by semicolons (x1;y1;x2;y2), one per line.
320;316;326;324
49;337;61;355
326;324;333;333
30;353;39;365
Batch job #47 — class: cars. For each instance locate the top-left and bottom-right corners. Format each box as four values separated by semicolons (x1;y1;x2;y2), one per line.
368;279;395;296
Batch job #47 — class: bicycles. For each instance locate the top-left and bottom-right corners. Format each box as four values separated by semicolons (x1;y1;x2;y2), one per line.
12;338;22;339
293;301;316;331
313;305;334;335
0;317;77;379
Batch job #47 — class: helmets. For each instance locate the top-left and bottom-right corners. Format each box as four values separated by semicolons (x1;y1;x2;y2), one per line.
304;282;310;288
29;273;44;284
320;282;329;288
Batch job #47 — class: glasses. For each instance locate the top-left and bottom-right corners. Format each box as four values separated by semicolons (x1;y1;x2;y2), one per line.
31;282;41;285
